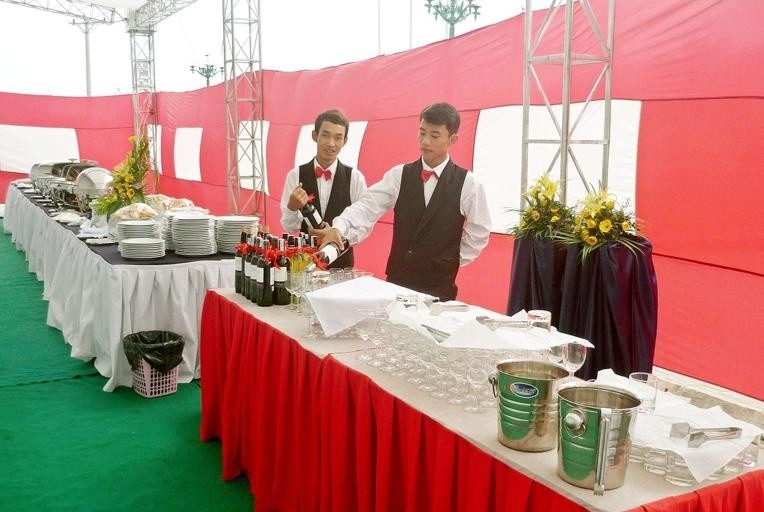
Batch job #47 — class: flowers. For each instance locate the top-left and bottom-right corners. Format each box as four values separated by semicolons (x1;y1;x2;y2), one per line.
510;174;575;247
547;182;652;270
88;128;155;220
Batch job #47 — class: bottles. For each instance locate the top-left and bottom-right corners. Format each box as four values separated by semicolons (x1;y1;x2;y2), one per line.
300;203;325;229
306;236;350;272
236;232;317;307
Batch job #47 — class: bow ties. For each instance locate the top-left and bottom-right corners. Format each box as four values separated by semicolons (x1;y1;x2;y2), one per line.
421;170;440;183
316;167;332;181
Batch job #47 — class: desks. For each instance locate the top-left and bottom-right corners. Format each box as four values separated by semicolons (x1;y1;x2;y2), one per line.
200;267;764;512
1;178;238;392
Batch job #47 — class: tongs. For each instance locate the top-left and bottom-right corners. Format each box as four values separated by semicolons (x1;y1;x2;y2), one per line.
421;324;451;343
476;316;531;331
429;303;470;316
670;423;742;448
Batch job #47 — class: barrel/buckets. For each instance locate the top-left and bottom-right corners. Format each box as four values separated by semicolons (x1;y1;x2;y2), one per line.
488;358;574;454
555;384;643;497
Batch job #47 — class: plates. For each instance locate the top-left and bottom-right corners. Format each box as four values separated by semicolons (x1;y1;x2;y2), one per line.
120;239;165;258
172;215;218;256
85;238;117;245
161;211;205;250
118;221;160;239
215;215;260;252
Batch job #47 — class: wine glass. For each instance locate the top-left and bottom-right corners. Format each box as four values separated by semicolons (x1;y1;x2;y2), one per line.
463;341;586;414
286;267;373;341
355;308;418;406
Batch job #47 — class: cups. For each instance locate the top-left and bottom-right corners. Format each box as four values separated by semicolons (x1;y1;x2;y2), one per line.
418;344;463;406
396;294;418;308
743;435;760;467
666;449;743;488
628;372;659;413
528;310;552;335
629;444;666;475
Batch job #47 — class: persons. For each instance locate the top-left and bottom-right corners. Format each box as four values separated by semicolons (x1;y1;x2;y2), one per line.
279;108;370;272
317;102;491;303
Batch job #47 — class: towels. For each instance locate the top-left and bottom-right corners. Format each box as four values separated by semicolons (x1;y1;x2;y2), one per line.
53;212;112;241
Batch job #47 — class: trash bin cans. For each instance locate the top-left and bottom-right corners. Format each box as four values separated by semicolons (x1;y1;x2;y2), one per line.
122;330;185;398
